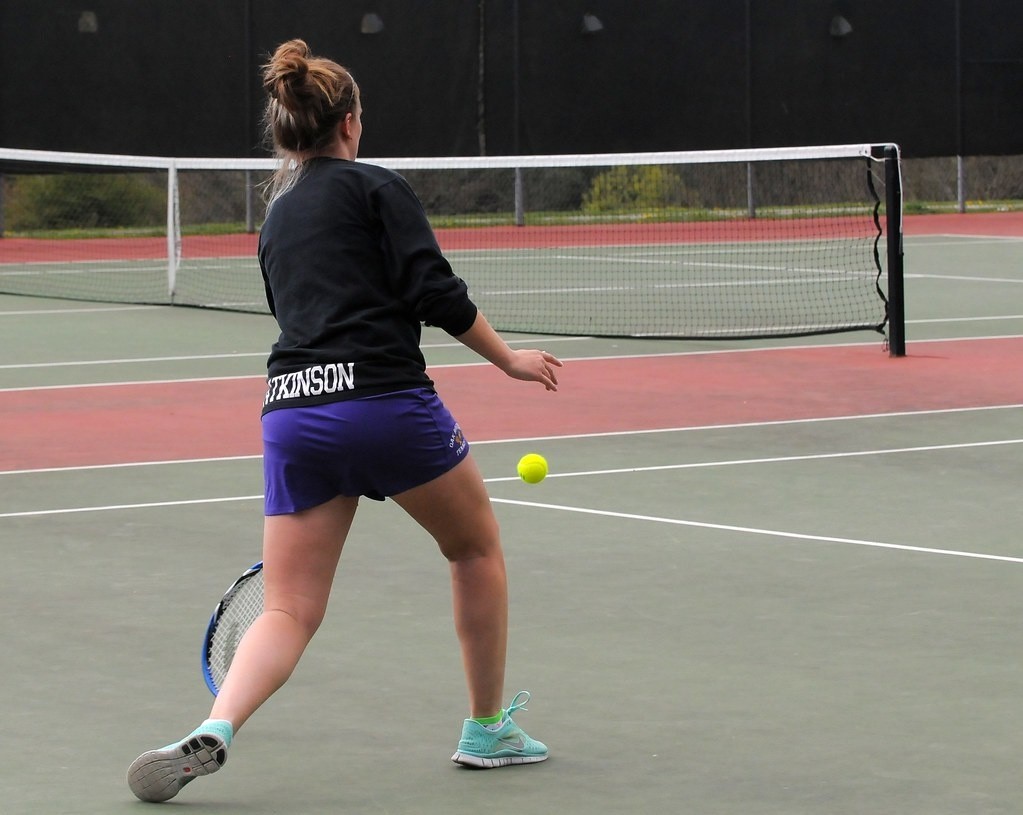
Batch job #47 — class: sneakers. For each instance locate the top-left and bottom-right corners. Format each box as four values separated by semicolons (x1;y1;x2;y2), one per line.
126;722;232;802
452;691;548;767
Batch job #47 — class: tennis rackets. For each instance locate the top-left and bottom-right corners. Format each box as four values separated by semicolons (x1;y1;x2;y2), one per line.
200;559;265;698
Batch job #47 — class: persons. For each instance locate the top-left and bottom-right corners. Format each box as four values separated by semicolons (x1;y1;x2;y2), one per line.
126;41;562;804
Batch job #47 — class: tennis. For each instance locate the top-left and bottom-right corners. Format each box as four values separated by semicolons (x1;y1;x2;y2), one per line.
516;452;549;485
